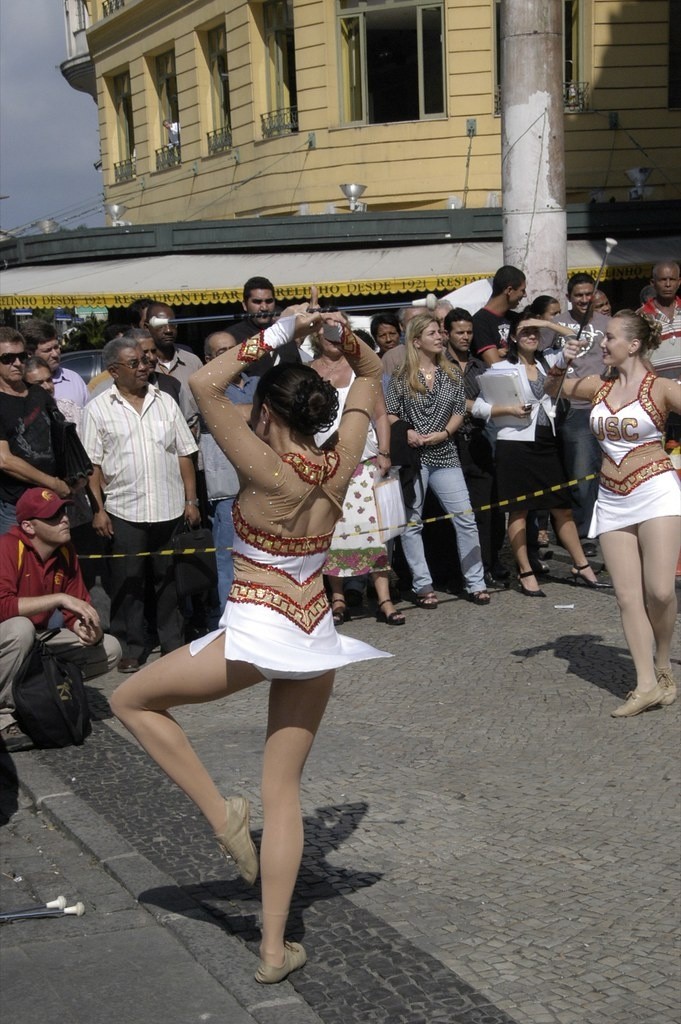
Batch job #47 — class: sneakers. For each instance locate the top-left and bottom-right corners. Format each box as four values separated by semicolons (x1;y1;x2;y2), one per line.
254;940;307;986
610;685;664;718
215;796;259;889
652;660;677;707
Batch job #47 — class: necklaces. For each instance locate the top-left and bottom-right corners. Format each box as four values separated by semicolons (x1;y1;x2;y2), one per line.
424;368;433;380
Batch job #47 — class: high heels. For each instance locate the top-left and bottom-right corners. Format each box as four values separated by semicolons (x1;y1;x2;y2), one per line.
570;563;613;588
375;599;405;624
467;591;490;604
519;572;546;597
412;592;438;609
330;598;350;625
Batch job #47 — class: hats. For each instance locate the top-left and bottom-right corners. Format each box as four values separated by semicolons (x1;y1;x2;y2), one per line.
16;488;75;525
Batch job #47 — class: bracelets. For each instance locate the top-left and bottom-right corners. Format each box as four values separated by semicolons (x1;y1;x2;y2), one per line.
185;498;200;507
379;452;391;458
445;429;450;438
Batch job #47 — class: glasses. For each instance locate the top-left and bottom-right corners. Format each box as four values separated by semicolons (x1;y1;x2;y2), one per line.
108;357;148;369
0;351;29;364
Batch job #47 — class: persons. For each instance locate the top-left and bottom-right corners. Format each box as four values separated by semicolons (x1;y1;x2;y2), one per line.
0;260;681;673
543;309;681;718
162;119;179;148
0;487;123;752
109;284;394;985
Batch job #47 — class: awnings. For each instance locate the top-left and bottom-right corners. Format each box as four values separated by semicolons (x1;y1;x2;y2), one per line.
0;239;681;309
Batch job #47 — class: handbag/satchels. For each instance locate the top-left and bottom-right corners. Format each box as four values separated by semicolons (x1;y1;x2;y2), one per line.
49;405;94;483
12;628;92;747
371;465;406;543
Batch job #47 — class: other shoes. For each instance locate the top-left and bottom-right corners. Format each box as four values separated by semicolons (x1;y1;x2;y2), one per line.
118;658;138;673
366;587;400;600
582;543;597;555
343;590;362;606
517;560;550;572
538;548;553;560
538;530;549;546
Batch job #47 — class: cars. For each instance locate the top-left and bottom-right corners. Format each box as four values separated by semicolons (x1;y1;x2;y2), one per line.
59;349;107;385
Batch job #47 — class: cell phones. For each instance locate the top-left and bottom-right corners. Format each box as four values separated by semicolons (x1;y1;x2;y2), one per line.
523;403;532;413
